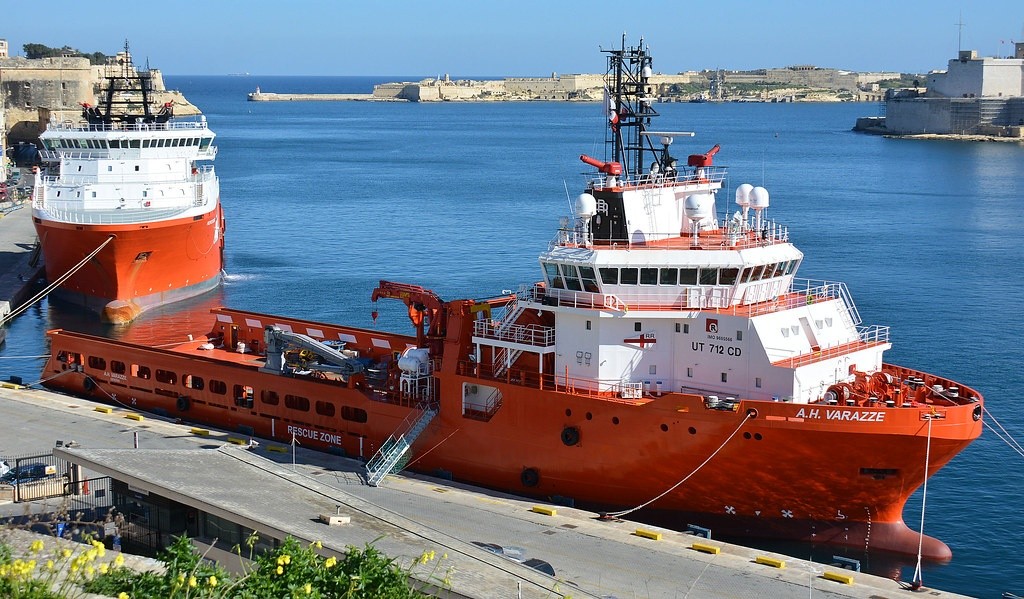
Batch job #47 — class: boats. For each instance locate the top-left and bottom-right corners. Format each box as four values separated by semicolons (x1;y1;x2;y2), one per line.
41;33;984;564
32;38;226;324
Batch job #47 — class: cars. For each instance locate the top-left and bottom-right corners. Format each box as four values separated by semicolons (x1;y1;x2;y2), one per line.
0;463;56;485
521;558;555;575
5;179;14;185
32;166;38;174
0;182;7;187
472;541;504;554
15;187;24;197
23;186;32;192
12;177;20;184
0;459;9;477
0;190;8;201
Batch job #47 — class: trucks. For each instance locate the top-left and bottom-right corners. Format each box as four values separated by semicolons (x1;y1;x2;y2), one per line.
49;522;104;545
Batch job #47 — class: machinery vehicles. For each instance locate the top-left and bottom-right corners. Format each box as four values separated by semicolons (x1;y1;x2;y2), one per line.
255;321;368;383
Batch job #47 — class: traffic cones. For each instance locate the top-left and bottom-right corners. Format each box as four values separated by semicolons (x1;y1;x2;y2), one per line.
84;479;91;494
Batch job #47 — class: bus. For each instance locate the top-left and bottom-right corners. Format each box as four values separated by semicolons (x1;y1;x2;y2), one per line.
12;168;20;179
